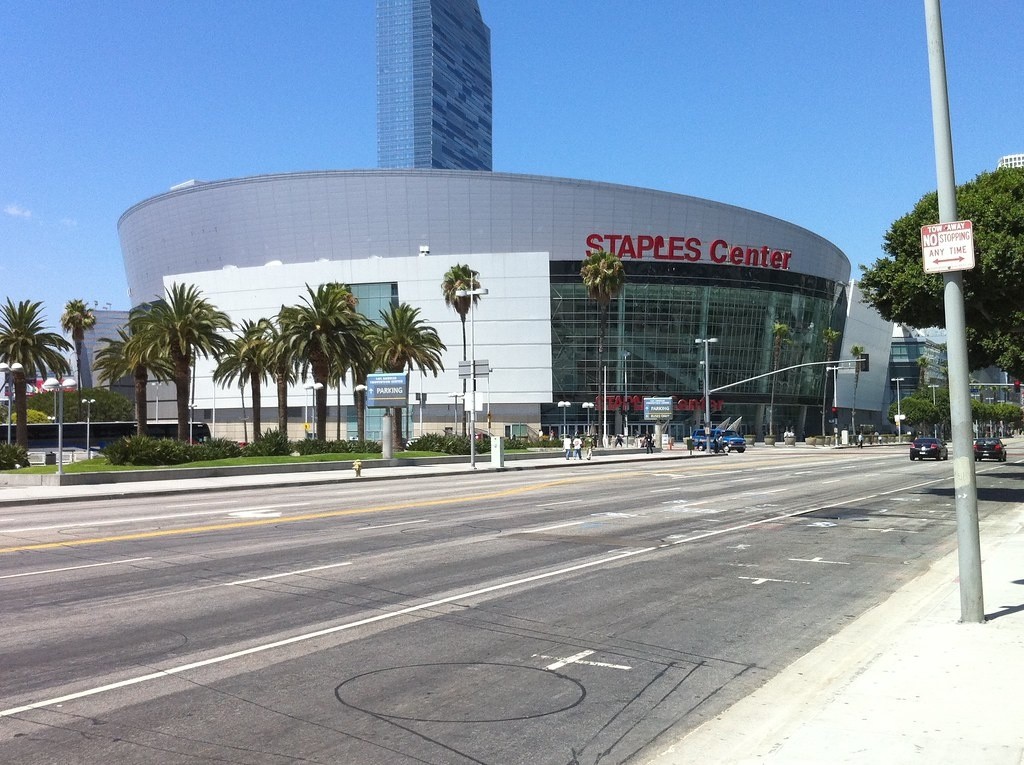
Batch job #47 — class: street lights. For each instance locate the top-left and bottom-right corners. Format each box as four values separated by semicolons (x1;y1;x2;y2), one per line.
928;384;940;438
695;337;719;453
44;377;78;474
210;370;218;439
448;394;464;435
558;401;572;439
304;383;324;441
970;394;1005;438
0;362;23;445
82;398;96;460
456;289;489;468
188;404;197;447
582;402;594;436
151;381;162;423
890;377;904;442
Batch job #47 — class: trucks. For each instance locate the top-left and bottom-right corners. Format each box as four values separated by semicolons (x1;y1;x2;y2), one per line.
692;429;745;454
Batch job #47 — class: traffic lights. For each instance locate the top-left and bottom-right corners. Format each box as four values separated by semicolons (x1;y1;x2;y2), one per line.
1014;379;1020;394
832;407;838;418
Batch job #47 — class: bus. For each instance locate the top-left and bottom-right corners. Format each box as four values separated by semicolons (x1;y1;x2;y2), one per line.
0;421;211;464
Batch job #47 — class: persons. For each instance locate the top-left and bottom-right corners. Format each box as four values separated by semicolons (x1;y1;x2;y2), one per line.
857;432;864;448
563;433;725;460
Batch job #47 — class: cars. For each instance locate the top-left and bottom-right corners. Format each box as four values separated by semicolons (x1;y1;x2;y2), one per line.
402;437;421;450
467;432;489;441
910;437;948;461
973;438;1007;462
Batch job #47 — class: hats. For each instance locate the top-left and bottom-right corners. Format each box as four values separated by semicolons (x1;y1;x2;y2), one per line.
587;434;590;436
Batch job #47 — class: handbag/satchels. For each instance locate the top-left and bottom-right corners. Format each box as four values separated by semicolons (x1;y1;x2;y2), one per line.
713;440;717;444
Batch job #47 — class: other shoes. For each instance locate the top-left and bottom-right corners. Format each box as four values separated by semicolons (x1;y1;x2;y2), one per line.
587;458;590;460
566;458;569;460
647;452;649;454
579;458;582;460
722;451;725;453
574;458;576;460
651;451;653;454
